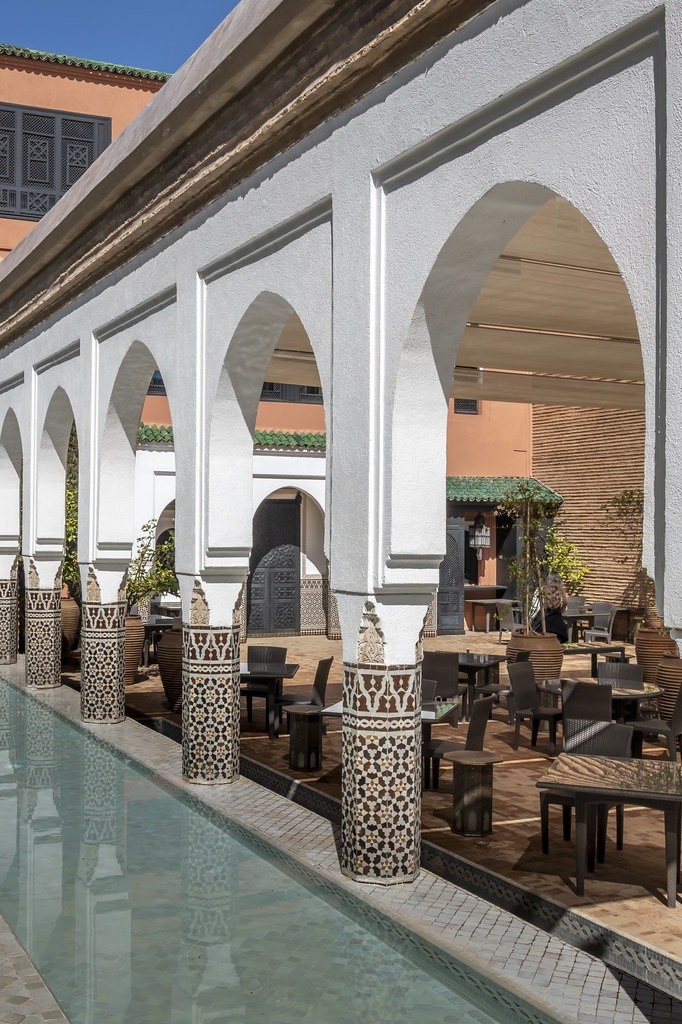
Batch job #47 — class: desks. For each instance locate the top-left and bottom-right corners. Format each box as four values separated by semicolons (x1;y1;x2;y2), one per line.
319;698;462;786
536;748;682;910
238;663;301;732
561;610;611;644
139;612;173;667
535;674;666;760
435;648;508;721
464;598;520;634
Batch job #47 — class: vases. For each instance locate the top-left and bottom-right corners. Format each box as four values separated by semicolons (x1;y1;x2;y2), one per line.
632;615;646;644
635;627;680;712
648;616;662;628
654;652;682;722
157;628;183;713
644;606;659;617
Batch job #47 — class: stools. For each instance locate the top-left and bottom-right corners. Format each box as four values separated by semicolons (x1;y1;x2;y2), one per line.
280;704;327;772
441;749;504;838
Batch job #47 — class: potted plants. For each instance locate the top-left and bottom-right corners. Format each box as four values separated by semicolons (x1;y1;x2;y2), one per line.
62;420;81;669
489;477;570;682
124;514;177;686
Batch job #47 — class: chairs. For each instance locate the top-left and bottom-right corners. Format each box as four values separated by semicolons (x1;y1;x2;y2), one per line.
239;592;682;873
